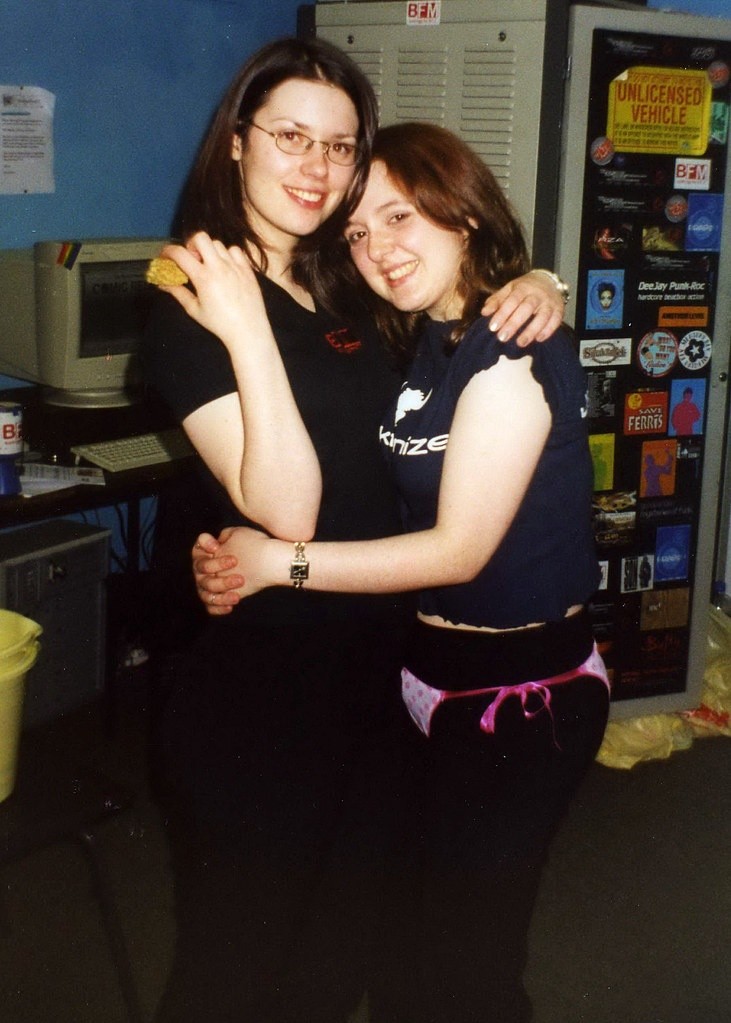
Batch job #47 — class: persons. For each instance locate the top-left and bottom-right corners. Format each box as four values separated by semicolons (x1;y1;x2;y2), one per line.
124;37;616;1023
597;282;616;310
670;387;700;435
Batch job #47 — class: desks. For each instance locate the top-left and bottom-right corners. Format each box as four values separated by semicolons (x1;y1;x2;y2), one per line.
1;384;198;530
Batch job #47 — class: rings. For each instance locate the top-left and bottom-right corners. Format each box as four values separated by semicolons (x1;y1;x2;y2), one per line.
212;593;215;605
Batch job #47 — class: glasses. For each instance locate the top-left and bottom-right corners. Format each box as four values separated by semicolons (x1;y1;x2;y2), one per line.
239;119;365;166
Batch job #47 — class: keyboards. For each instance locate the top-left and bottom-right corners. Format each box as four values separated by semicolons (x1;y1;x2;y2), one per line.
70;429;198;472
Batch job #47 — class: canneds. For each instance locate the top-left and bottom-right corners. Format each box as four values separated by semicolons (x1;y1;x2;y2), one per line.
0;401;23;495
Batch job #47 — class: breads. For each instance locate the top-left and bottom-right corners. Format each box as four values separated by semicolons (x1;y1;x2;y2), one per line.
146;258;189;286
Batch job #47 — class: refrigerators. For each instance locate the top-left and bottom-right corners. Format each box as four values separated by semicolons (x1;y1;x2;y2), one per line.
290;1;730;729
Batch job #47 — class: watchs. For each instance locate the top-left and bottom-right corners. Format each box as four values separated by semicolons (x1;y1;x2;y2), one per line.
290;542;309;589
530;268;570;306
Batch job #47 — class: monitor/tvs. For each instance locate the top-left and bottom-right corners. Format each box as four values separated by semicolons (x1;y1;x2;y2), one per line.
0;237;177;409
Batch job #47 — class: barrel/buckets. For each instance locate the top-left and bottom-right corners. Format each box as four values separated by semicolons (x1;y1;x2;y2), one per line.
1;610;44;806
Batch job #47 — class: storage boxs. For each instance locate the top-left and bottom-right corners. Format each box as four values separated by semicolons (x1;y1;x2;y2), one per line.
0;520;114;732
